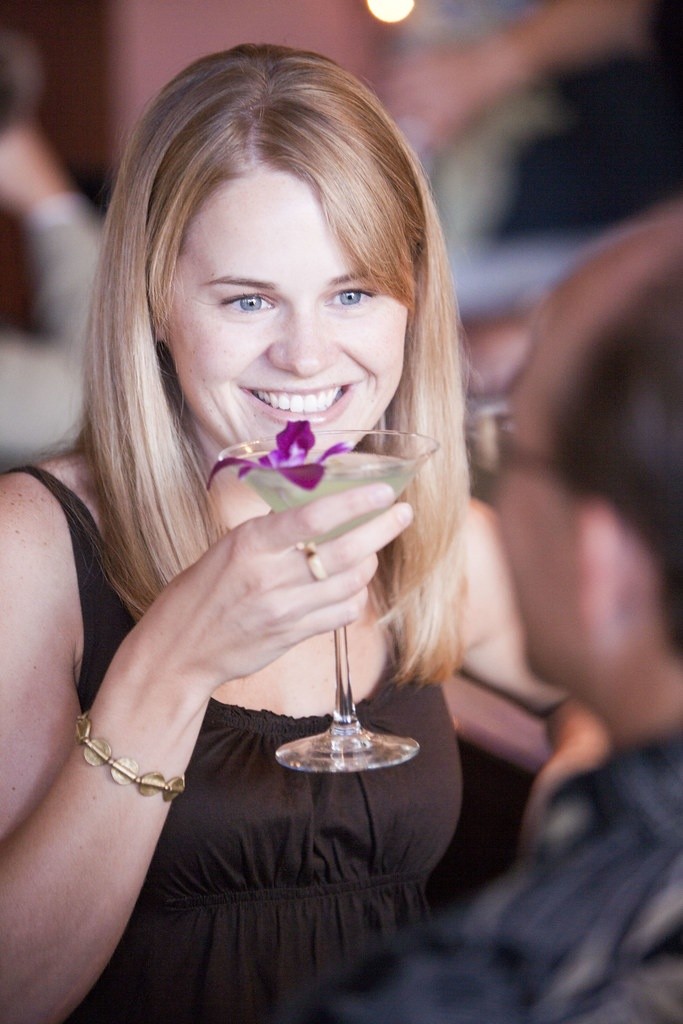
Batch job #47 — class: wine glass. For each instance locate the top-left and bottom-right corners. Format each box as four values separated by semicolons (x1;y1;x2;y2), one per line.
217;430;441;773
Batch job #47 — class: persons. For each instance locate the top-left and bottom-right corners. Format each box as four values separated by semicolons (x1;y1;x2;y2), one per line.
0;0;683;1024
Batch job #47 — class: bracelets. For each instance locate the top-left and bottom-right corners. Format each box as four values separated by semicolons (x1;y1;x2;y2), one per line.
73;711;186;803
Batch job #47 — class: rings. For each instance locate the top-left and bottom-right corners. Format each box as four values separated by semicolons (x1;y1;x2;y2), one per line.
296;540;328;581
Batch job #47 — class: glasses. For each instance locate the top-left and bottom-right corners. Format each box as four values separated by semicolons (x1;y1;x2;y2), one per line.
473;404;617;500
